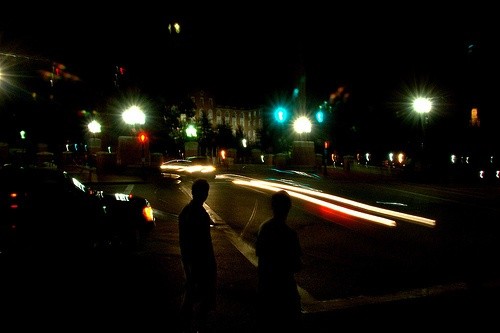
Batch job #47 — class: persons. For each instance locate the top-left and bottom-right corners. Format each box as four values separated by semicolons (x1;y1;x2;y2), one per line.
256;190;304;333
331;150;337;167
178;178;218;333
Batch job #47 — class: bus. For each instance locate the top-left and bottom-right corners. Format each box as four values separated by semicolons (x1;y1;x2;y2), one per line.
118;135;152;172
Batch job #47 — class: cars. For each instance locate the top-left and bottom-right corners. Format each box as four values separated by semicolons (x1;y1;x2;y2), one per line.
0;164;155;264
158;158;217;180
